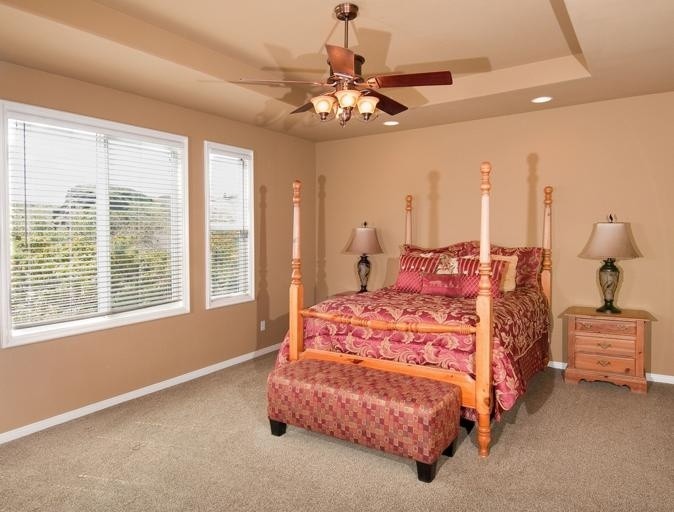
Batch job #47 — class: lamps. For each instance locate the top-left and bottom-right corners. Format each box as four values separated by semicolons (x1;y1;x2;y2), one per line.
578;216;642;313
346;221;384;294
310;80;381;125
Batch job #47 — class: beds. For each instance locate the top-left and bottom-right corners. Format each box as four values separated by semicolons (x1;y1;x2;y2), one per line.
274;160;554;458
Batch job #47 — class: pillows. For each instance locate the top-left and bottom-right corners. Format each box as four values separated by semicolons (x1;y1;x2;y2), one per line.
410;250;458;275
416;271;465;299
458;256;505;300
396;254;439;292
470;255;518;292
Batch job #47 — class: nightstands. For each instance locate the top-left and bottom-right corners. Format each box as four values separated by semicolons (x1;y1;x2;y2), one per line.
564;307;653;392
333;290;373;297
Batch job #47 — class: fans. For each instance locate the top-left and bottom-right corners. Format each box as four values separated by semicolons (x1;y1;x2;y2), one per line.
228;44;453;116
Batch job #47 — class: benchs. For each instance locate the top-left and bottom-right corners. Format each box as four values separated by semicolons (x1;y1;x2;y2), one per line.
266;359;461;481
